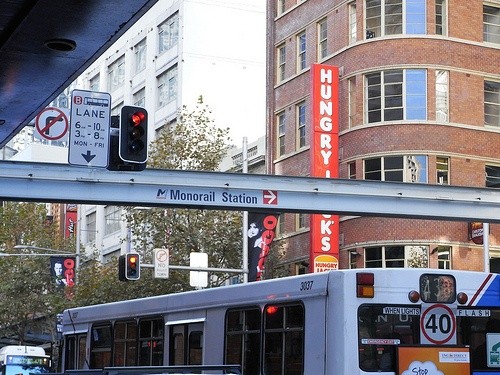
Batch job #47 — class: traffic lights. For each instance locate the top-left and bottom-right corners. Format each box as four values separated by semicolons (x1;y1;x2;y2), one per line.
119;254;140;282
119;105;150;163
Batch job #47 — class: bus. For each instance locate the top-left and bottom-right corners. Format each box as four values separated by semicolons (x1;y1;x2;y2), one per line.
0;345;54;375
57;267;500;375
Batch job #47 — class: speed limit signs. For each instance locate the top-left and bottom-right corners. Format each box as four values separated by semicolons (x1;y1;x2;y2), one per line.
420;301;458;347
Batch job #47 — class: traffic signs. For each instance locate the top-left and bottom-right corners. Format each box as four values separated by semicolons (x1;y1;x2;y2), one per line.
68;91;110;169
